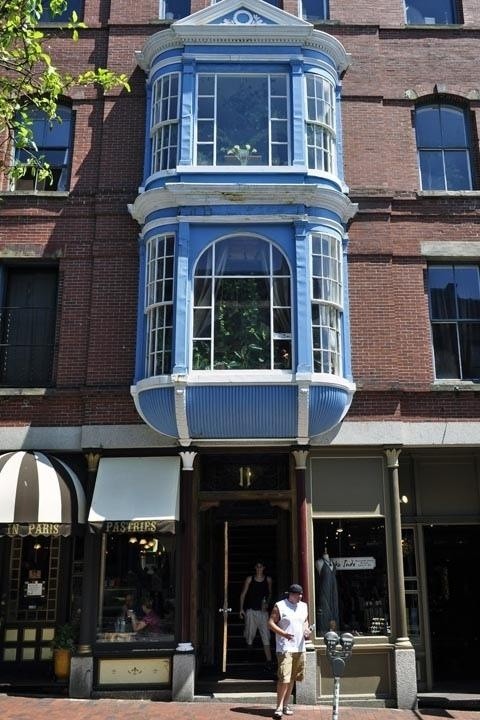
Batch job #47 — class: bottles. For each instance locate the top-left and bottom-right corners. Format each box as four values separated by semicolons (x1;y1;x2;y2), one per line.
121;619;126;633
105;575;128;587
261;596;267;611
308;623;315;633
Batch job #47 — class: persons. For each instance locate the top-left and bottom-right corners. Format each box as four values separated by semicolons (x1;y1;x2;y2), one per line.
268;583;316;717
120;593;135;618
126;599;161;634
240;561;273;671
316;553;339;634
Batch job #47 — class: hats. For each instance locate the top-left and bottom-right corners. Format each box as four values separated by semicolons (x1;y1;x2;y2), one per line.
285;584;303;594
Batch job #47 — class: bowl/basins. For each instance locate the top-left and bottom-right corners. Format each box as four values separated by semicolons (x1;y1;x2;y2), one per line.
178;642;192;647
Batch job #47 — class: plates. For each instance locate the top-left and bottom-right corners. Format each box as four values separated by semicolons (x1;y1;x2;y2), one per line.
176;646;194;651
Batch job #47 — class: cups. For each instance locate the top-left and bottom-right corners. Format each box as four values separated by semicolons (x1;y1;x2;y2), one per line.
127;610;133;617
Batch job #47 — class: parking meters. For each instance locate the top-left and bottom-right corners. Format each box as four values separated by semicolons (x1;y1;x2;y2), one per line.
324;632;355;720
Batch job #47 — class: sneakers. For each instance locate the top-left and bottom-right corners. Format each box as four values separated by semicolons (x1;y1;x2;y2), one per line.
283;706;293;714
274;708;283;717
